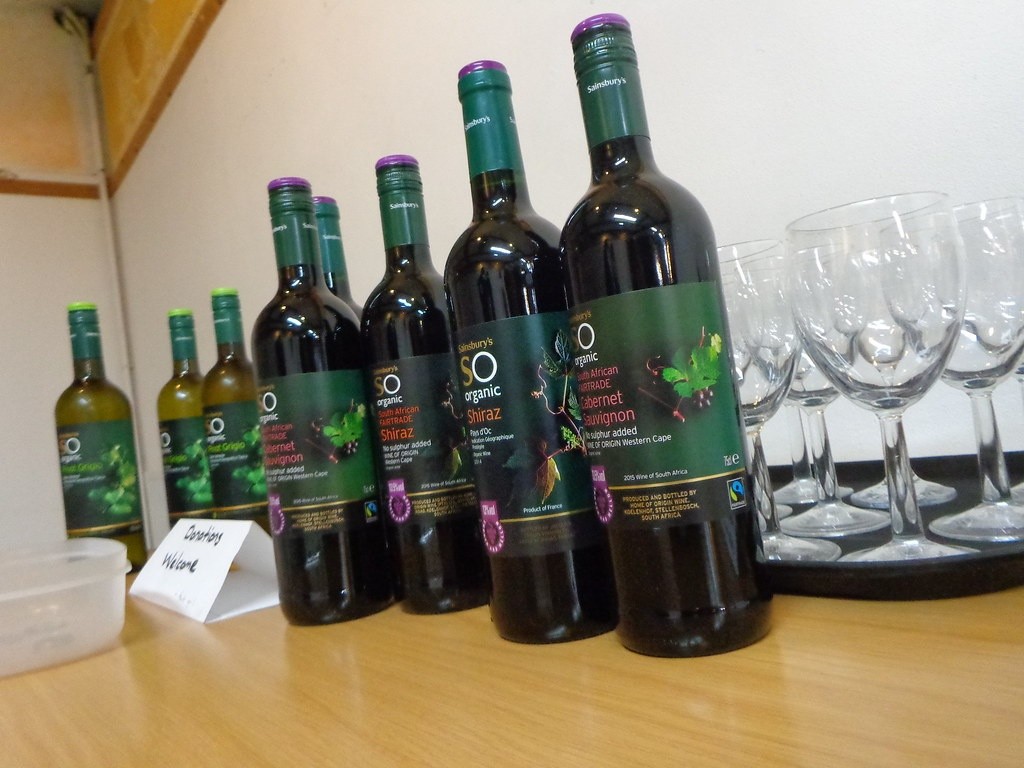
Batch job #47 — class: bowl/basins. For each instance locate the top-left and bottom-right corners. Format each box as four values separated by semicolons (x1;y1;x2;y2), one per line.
0;535;132;679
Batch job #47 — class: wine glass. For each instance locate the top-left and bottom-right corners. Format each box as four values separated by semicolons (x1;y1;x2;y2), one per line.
711;190;1023;563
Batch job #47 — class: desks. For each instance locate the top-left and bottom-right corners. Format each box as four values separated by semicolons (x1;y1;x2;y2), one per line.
0;561;1023;767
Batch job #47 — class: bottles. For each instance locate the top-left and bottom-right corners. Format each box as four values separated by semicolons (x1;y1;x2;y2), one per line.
156;308;213;532
201;287;270;547
249;177;407;627
358;153;496;615
560;13;770;656
309;195;364;328
438;59;621;647
54;300;148;575
